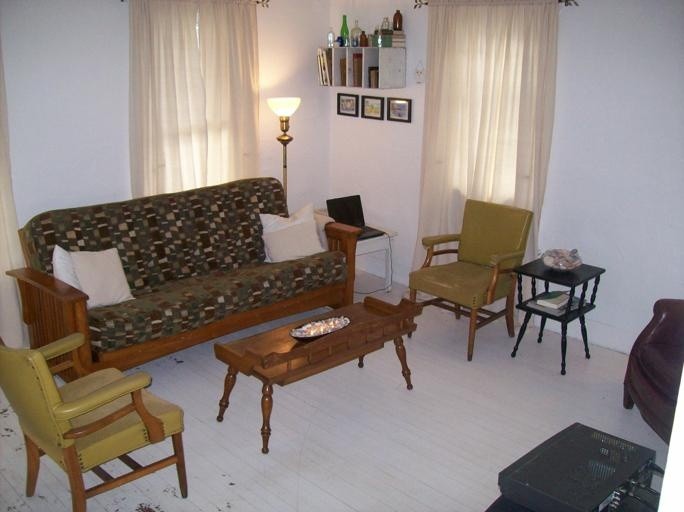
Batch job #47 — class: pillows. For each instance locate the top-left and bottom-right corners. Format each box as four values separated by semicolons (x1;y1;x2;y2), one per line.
53;243;135;309
260;201;325;262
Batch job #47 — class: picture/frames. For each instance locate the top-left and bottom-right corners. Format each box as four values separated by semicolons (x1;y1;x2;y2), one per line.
388;98;411;123
361;96;383;119
337;92;359;117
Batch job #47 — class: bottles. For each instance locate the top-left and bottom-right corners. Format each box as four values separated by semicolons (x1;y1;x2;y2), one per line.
328;10;402;47
369;66;378;88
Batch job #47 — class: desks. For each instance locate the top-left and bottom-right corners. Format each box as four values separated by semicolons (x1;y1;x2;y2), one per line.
513;260;605;375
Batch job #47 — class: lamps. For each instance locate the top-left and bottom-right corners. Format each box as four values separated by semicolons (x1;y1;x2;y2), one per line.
267;96;300;215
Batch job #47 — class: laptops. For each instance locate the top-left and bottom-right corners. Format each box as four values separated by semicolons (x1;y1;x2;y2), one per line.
326;194;385;242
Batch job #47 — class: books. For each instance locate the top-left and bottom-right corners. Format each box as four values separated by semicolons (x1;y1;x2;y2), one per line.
317;30;406;87
527;291;586;317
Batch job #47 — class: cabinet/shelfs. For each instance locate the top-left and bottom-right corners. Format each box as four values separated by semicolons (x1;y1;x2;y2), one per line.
317;48;417;96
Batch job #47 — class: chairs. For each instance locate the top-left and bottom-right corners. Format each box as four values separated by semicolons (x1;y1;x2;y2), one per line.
622;299;683;445
0;333;188;512
408;199;533;361
326;194;399;298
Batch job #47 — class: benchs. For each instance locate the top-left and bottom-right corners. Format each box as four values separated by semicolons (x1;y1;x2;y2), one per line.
5;177;362;383
